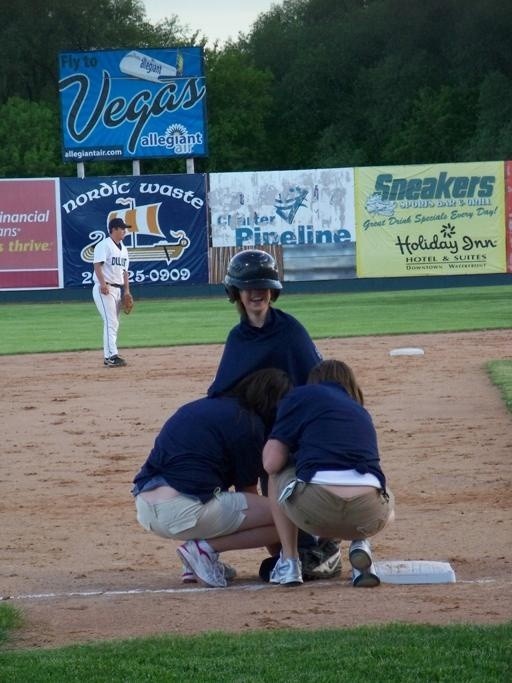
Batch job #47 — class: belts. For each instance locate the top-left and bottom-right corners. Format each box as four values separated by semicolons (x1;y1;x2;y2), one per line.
106;283;121;287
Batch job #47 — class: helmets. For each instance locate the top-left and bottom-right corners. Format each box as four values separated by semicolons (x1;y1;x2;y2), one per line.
223;249;283;303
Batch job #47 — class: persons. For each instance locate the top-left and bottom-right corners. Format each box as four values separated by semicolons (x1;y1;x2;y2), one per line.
130;366;294;587
261;360;395;587
208;248;344;578
92;218;133;368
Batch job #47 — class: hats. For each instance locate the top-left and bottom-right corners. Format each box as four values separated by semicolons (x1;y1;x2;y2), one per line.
109;219;131;229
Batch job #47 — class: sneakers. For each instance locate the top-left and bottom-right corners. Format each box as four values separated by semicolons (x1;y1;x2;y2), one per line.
349;539;380;586
104;356;126;366
259;540;342;585
176;539;236;587
